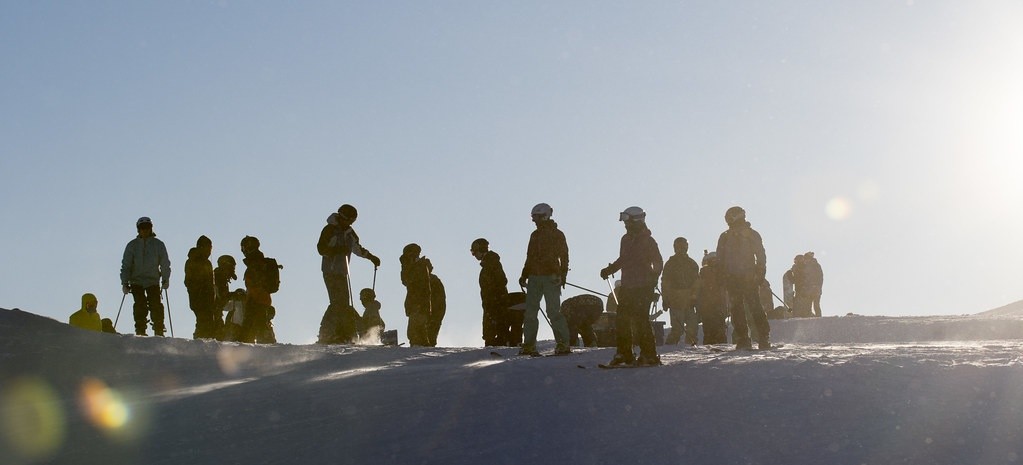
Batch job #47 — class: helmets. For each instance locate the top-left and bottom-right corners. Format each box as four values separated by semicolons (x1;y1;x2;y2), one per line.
675;237;688;253
361;288;375;299
137;217;154;231
726;206;746;227
338;205;357;218
241;236;260;253
623;207;645;223
704;252;717;259
531;204;552;221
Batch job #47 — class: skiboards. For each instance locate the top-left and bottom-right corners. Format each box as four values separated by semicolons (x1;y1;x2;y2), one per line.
490;351;558;357
579;364;644;368
711;344;784;351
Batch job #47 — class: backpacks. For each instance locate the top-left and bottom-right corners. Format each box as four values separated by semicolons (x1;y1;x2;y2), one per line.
264;257;282;292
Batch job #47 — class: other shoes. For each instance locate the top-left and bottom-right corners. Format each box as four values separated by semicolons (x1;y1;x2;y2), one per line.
155;329;164;336
520;348;537;355
636;355;660;366
758;341;770;350
735;341;752;351
609;352;635;365
554;345;570;353
135;331;148;336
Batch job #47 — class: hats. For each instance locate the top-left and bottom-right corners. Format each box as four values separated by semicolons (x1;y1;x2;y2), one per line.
218;255;236;267
402;244;421;255
197;235;211;247
469;238;488;252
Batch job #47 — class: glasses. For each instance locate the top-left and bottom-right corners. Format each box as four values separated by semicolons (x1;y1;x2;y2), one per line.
619;212;645;220
725;211;745;223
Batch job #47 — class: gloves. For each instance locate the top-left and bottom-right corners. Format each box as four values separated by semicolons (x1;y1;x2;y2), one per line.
519;276;527;287
663;301;669;311
121;286;130;293
160;280;169;290
600;267;609;280
559;275;566;285
371;255;380;266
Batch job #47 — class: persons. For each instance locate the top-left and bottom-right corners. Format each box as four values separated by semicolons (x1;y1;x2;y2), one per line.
427;260;446;347
698;252;729;345
318;288;385;345
101;318;117;334
508;291;526;347
184;235;216;338
470;238;508;345
518;203;570;354
317;204;380;344
69;293;103;332
601;206;664;367
562;279;623;347
716;206;772;349
400;243;433;346
240;236;278;344
224;288;247;341
120;217;171;337
661;237;702;344
760;251;823;317
214;255;238;340
